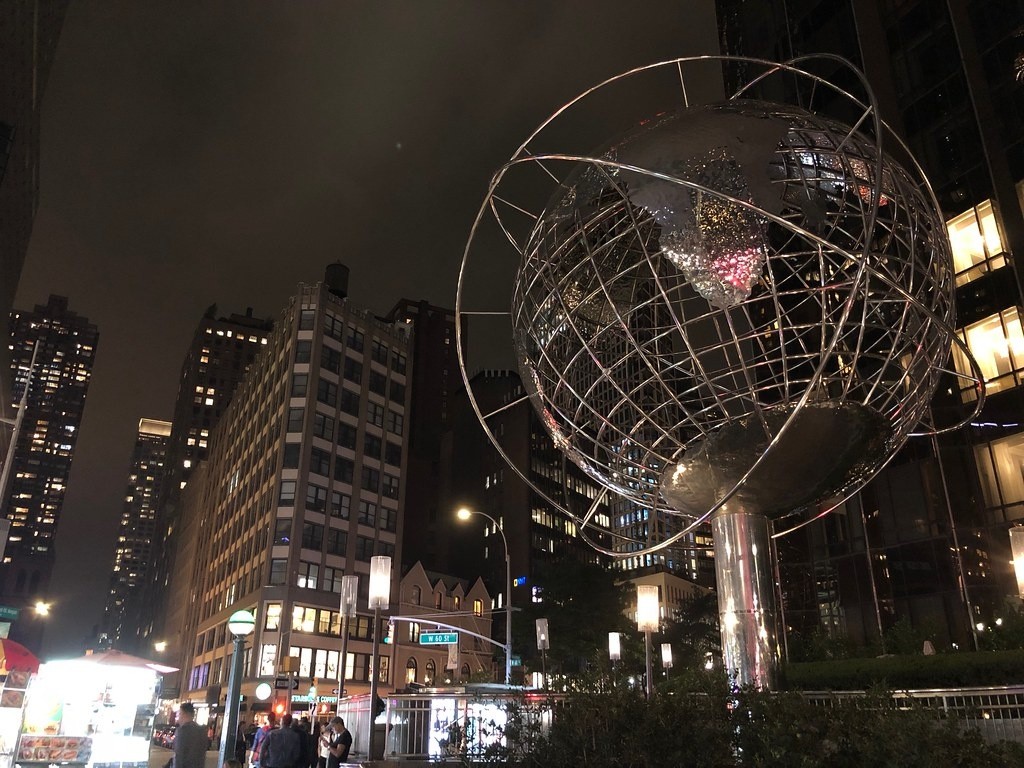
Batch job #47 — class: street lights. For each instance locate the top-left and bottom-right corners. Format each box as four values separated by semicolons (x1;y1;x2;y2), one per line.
636;584;661;703
660;643;673;681
367;554;393;760
455;505;515;683
212;609;257;768
336;573;359;699
608;631;623;689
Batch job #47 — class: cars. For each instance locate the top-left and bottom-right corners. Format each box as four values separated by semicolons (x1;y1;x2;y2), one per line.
152;723;213;752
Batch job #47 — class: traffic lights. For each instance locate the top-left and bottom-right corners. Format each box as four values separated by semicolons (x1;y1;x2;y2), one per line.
312;676;319;688
386;624;394;645
318;702;329;714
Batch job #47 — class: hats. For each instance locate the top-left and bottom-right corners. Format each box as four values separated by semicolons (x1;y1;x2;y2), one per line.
326;717;343;728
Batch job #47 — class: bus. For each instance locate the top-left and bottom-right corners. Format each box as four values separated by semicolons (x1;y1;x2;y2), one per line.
253;712;310;728
302;712;336;727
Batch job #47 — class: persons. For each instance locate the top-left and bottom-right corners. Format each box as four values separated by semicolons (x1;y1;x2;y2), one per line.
234;713;352;768
171;703;206;768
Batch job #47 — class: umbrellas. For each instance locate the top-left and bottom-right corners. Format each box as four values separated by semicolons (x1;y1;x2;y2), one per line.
0;638;40;676
